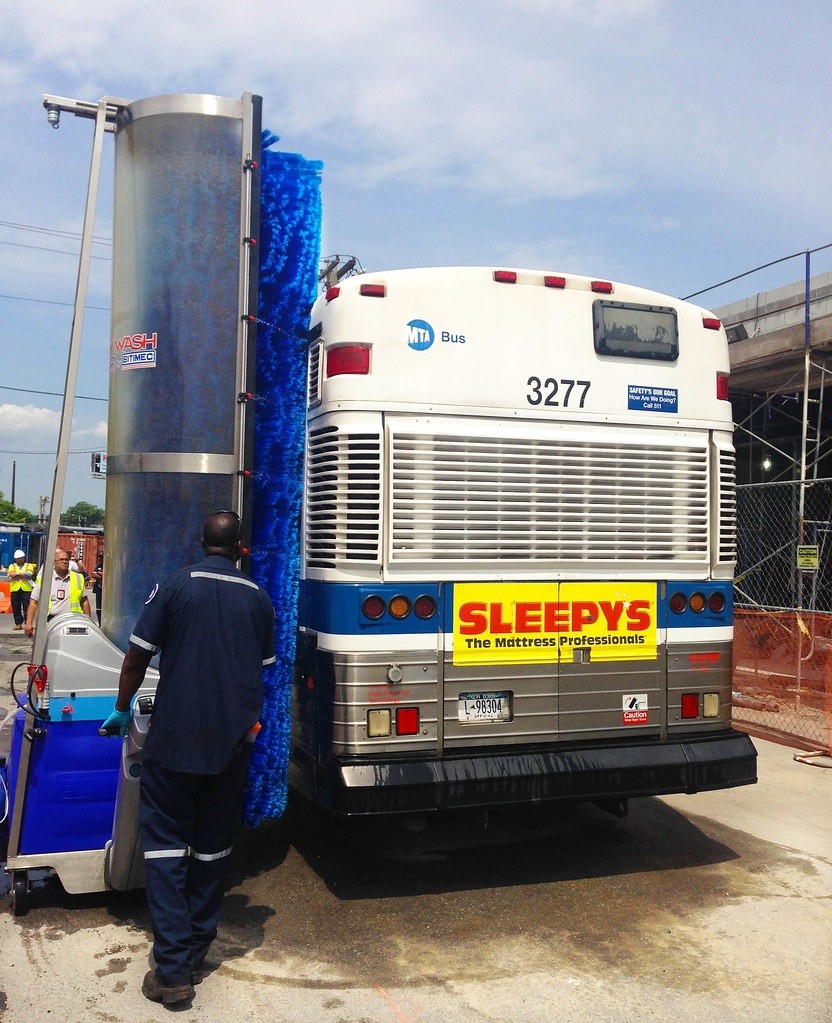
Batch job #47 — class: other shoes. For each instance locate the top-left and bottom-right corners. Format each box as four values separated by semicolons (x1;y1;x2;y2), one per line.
154;943;202;985
142;970;196;1004
13;624;22;630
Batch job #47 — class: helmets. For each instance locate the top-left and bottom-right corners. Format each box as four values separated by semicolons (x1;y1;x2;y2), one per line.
96;550;104;557
14;550;25;558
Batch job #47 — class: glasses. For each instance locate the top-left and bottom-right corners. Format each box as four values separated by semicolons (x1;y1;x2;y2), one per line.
214;509;241;551
55;558;69;563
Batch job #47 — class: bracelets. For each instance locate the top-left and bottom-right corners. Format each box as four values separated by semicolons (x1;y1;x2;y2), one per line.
75;558;79;562
16;573;19;575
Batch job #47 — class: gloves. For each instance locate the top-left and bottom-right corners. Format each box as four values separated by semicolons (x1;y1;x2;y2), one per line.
101;707;132;737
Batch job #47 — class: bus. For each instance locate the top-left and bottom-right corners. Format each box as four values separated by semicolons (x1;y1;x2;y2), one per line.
289;254;757;824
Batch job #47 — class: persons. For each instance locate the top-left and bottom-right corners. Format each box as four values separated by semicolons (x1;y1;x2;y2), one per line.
92;551;104;627
102;512;277;1003
8;550;34;630
24;546;91;638
